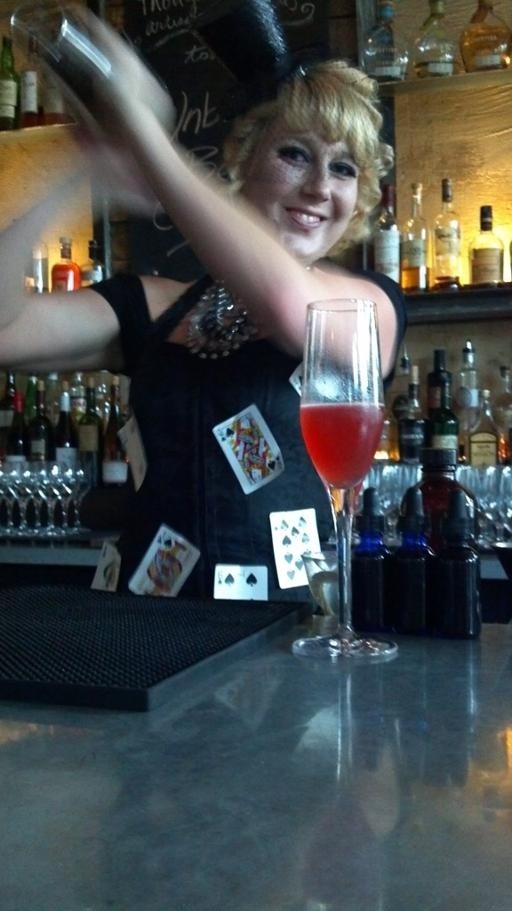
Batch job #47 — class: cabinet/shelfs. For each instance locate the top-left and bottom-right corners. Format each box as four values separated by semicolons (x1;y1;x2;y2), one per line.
0;0;132;566
329;0;511;556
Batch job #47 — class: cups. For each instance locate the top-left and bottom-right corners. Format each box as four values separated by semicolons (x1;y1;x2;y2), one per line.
301;552;339;633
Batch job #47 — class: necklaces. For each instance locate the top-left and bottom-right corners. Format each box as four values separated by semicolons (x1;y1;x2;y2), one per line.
186;280;261;361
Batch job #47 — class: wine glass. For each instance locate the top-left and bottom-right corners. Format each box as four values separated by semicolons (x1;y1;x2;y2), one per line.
290;297;400;668
356;463;512;554
0;463;92;539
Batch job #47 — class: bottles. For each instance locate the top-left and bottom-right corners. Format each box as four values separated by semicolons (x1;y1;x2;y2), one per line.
349;446;484;641
431;176;462;290
458;0;512;72
78;238;105;288
0;366;86;471
0;30;18;127
43;51;69;127
412;0;461;79
51;235;81;292
373;182;401;285
76;375;105;489
358;0;411;81
388;336;511;471
399;181;430;296
468;203;505;285
23;231;49;295
101;374;128;486
17;26;42;130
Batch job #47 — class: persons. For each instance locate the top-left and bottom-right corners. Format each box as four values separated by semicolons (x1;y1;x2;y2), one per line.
0;0;408;604
